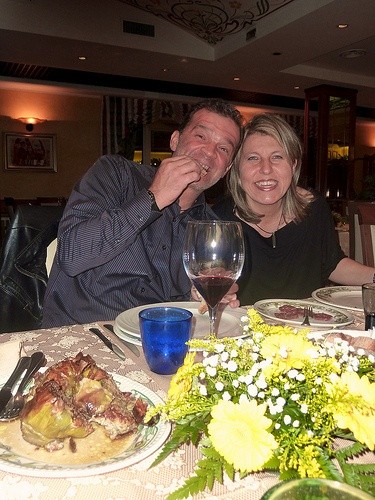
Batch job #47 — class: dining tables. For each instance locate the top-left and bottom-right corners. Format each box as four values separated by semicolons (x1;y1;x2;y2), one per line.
0;296;375;500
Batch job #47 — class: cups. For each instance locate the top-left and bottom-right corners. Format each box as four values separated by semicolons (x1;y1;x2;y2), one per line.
362;282;375;331
261;479;375;500
138;306;194;376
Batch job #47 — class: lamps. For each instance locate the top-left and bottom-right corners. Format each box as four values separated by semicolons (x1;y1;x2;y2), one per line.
17;117;42;131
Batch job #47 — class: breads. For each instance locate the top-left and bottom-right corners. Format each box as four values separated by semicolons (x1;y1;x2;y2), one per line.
349;336;375;355
192;289;209;314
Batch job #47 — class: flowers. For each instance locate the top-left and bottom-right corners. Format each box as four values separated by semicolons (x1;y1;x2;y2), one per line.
144;308;375;500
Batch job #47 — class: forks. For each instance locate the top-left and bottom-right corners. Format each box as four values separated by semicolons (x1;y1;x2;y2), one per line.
301;306;313;326
0;352;45;421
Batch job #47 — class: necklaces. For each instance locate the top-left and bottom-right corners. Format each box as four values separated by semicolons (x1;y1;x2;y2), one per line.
181;210;187;211
256;213;282;248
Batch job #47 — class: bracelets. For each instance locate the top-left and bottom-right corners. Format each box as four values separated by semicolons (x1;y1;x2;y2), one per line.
147;191;159;211
373;273;375;283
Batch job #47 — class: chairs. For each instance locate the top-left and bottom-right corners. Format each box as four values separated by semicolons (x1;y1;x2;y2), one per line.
348;200;375;268
4;196;66;321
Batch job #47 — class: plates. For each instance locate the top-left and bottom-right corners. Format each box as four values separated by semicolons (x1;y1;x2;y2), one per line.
0;366;173;479
253;298;354;331
113;301;255;352
311;285;364;312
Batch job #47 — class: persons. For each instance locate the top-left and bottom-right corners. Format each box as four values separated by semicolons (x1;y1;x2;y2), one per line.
40;98;246;328
118;139;134;161
13;138;33;165
212;112;375;306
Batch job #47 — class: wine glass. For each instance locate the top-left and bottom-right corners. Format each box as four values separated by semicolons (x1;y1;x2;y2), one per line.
183;219;245;342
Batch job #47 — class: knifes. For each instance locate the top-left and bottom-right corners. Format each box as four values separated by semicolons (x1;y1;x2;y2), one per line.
89;328;126;361
103;324;140;357
0;356;31;412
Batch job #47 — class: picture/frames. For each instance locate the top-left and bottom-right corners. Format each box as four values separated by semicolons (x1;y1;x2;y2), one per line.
3;132;57;173
